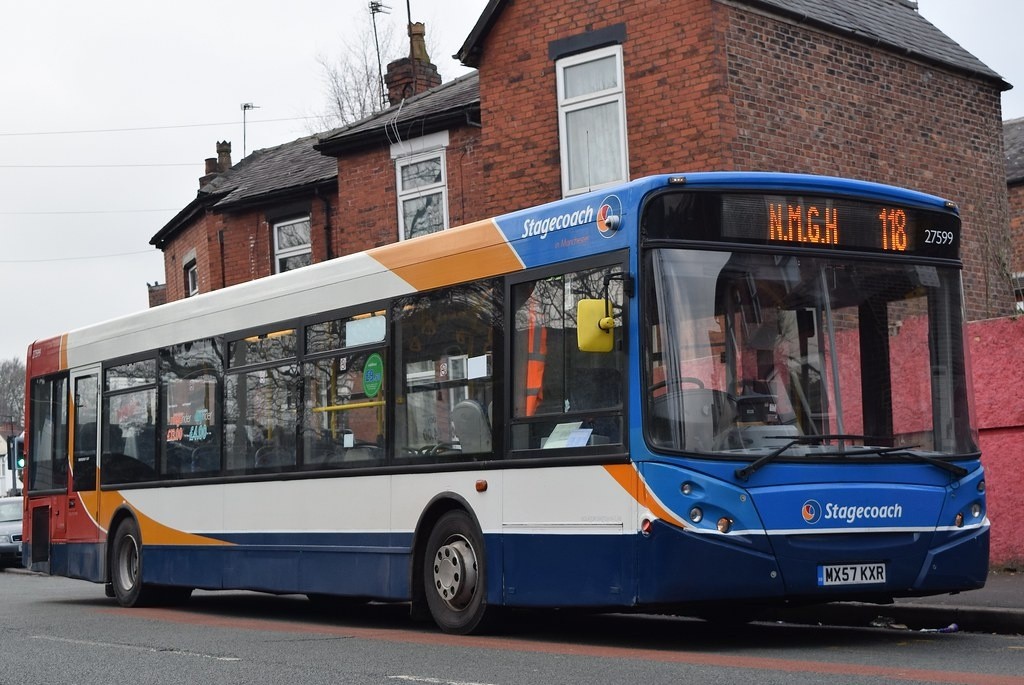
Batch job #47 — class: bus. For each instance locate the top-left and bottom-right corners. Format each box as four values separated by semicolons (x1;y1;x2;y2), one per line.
21;170;991;639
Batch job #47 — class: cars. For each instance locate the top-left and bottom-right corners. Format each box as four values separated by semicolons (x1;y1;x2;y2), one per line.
0;496;24;562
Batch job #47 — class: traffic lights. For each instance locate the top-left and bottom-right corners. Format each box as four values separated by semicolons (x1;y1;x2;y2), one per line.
14;436;26;470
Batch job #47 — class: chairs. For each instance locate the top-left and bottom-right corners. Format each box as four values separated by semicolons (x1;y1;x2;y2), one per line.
59;422;383;475
453;401;492;453
487;401;530;450
